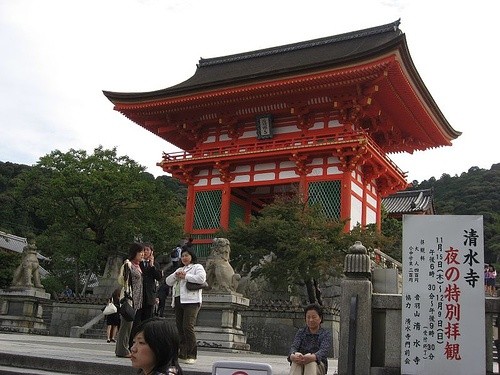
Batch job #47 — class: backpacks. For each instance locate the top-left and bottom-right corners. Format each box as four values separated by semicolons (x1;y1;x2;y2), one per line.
171;249;179;258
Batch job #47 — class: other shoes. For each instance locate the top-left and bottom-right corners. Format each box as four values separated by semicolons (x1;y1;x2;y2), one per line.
111;338;117;343
186;358;196;364
107;339;111;343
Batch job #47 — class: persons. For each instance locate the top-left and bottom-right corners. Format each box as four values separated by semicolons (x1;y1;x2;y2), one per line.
171;243;181;267
484;264;496;297
287;305;331;375
115;242;144;358
105;284;169;343
128;242;161;350
165;249;206;364
130;316;183;375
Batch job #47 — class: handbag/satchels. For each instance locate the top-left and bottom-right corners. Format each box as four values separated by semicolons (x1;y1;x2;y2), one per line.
103;297;118;315
119;296;136;322
186;281;209;290
